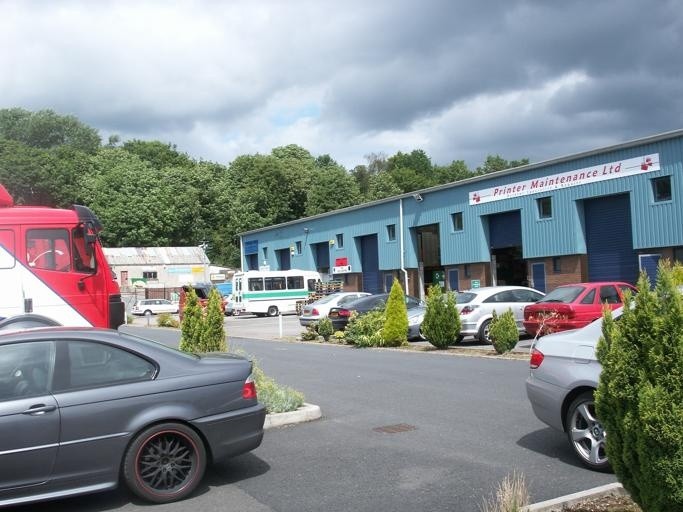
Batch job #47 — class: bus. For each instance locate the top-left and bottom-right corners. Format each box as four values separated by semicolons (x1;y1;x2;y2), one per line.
232;269;325;317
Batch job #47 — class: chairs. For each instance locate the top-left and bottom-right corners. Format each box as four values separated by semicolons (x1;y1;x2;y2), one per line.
5;345;140;396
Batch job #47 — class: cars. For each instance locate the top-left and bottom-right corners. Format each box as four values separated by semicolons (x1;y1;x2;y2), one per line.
132;297;179;316
523;281;641;339
523;286;683;475
406;303;430;342
222;293;233;315
298;291;373;331
327;292;425;332
0;330;269;504
442;285;547;345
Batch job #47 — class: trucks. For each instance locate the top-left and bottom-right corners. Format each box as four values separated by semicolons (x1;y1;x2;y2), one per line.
0;184;124;330
